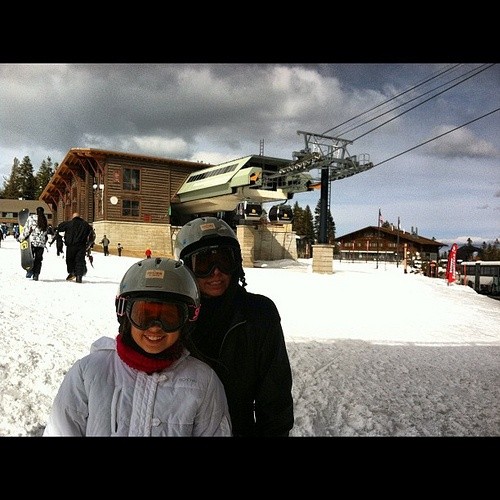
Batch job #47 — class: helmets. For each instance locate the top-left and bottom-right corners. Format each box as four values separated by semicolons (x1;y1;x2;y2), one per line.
174;217;240;260
119;257;199;305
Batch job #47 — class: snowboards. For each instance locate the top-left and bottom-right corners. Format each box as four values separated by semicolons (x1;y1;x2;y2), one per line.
19;209;34;272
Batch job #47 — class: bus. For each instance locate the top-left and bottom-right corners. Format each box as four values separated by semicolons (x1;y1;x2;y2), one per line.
460;261;500;293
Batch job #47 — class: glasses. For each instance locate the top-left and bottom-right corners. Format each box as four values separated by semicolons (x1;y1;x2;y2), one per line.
115;297;200;333
185;246;235;278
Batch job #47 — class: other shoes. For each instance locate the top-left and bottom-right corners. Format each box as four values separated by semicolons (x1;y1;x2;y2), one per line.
33;274;38;280
26;272;33;278
66;273;76;280
76;276;82;283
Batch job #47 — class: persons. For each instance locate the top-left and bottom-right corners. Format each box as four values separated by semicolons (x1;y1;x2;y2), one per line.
49;229;64;257
56;213;95;282
43;223;58;252
173;217;295;437
99;234;110;256
0;223;19;248
145;248;151;259
16;207;48;280
42;256;233;437
117;243;123;256
